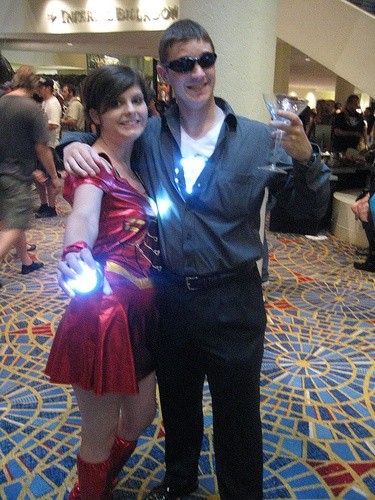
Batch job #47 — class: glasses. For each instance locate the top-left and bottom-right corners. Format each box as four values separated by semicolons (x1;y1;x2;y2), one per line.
160;52;217;72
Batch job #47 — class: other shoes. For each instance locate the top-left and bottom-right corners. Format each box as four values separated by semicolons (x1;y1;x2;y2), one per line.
23;260;43;275
34;203;48;213
35;206;57;218
355;247;369;256
354;260;375;273
27;242;36;251
143;478;200;499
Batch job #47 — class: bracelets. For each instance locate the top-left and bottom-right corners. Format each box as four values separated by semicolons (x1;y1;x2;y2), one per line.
62;241;93;260
307;147;318;163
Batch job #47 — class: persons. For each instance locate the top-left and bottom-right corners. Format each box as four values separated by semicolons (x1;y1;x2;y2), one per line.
271;95;375;150
0;64;160;288
352;160;375;273
44;65;159;500
55;20;331;500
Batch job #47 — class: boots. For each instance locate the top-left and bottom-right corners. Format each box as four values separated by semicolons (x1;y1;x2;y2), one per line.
69;437;143;500
75;452;113;500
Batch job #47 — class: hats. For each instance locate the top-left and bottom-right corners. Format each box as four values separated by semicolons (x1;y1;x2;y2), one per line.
38;76;54;87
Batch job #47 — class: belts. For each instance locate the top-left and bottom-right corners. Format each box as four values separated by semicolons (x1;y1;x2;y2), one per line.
164;261;258;290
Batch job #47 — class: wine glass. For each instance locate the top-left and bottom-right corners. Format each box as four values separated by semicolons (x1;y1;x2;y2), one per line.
258;92;309;174
